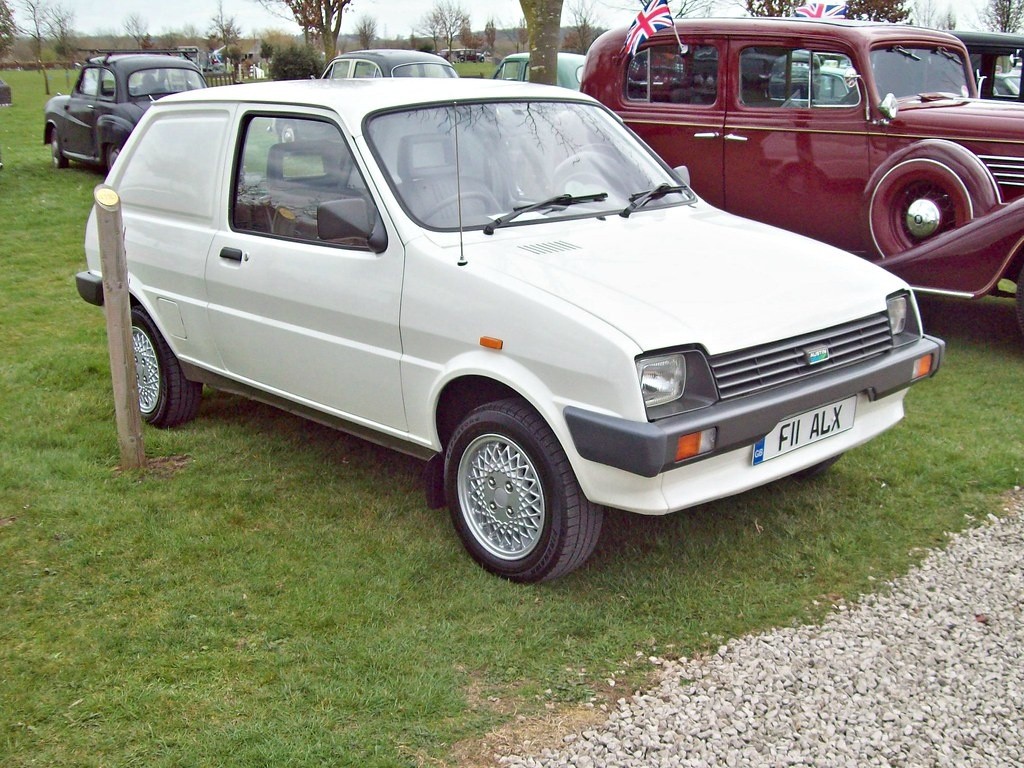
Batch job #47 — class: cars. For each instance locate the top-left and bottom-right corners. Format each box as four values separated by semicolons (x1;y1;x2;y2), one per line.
437;48;484;66
582;16;1023;338
274;49;460;148
629;31;1023;106
42;45;211;177
74;76;947;583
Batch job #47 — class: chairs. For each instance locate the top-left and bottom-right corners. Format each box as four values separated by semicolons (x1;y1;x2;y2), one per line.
395;133;499;220
316;199;372;248
101;88;115;97
258;141;353;231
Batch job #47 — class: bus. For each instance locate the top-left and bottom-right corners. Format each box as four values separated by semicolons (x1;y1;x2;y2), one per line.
493;52;586;95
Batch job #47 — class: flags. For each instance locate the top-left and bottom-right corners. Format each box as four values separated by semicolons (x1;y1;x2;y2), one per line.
624;0;673;54
794;0;846;19
